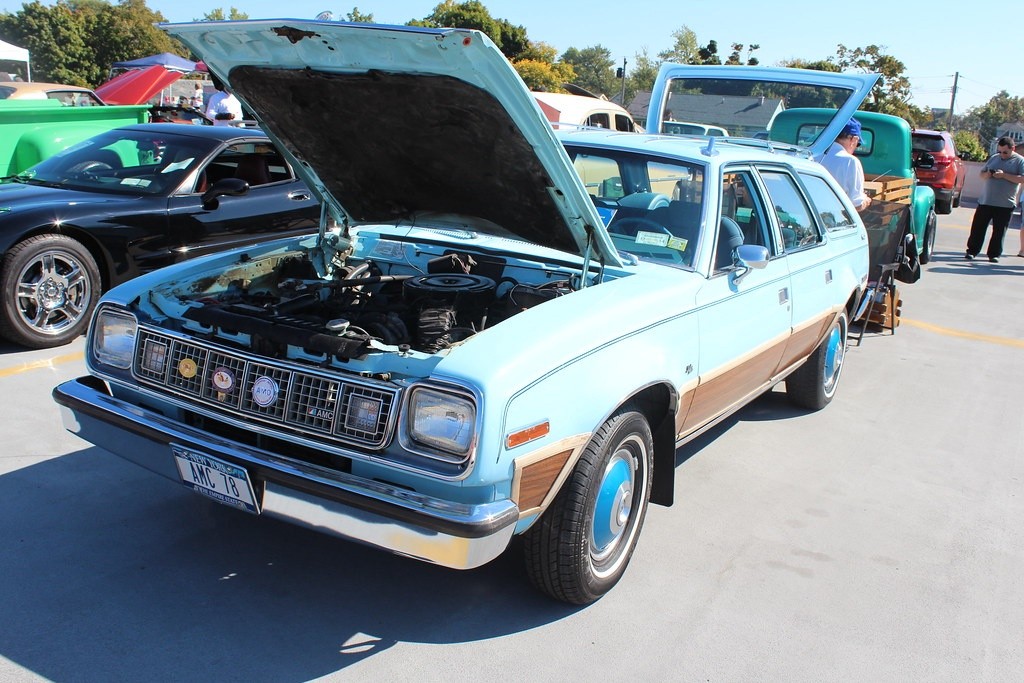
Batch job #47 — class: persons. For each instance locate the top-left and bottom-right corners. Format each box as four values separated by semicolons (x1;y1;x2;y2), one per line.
190;83;204;110
965;137;1024;263
814;117;873;213
206;86;246;127
661;110;678;135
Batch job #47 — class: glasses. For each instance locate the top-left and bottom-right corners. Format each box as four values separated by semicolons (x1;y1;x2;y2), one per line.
856;142;861;147
997;151;1008;154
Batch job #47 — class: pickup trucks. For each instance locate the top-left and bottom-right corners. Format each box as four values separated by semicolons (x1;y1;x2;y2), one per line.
1;97;154;183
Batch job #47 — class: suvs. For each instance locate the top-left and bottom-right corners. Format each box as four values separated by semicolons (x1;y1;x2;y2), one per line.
910;127;970;214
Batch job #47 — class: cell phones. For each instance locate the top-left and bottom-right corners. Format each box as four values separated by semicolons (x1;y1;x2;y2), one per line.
989;168;996;174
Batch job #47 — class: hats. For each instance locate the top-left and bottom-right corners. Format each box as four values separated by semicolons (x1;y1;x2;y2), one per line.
844;116;864;141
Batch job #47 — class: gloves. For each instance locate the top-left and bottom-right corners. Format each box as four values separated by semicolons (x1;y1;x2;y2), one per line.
215;113;235;120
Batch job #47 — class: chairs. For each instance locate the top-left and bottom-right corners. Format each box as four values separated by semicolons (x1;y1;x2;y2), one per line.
682;214;742;272
233;155;273;186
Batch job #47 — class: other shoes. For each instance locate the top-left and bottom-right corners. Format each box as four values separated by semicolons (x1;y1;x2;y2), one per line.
965;252;975;261
1017;252;1024;257
988;256;999;262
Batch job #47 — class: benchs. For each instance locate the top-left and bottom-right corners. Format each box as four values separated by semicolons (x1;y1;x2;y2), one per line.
736;219;797;254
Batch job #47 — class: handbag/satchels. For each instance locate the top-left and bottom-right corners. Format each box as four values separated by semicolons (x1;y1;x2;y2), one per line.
892;208;921;284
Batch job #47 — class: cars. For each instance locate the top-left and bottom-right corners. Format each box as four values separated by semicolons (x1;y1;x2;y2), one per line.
0;122;335;352
0;64;217;125
52;16;884;608
528;81;937;267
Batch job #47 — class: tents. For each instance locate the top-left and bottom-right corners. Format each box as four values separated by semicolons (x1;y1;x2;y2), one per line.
109;51;198;106
